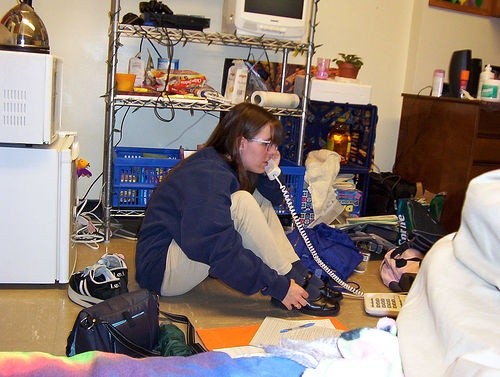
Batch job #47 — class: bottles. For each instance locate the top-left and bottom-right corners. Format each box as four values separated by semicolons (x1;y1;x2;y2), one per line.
476;64;495;100
128;52;145;87
224;60;249;104
327;116;352;165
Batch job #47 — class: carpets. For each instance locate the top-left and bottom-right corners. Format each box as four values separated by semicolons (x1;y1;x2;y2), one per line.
196;315;352;351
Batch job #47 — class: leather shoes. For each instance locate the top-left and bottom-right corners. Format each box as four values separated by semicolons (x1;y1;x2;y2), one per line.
304;271;344;302
269;281;339;318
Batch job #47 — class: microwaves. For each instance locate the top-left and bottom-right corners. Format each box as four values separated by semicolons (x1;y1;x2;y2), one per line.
0;51;59;147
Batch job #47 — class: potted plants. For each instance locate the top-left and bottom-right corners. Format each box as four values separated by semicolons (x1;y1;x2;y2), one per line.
336;51;363;78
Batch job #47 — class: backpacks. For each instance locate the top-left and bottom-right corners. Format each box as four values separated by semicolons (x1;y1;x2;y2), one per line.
377;241;427;293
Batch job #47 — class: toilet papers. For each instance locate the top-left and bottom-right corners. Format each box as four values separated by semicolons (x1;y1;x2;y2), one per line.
250;90;300;109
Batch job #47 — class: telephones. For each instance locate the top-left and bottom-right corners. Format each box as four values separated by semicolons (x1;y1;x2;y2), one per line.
264;160;280;181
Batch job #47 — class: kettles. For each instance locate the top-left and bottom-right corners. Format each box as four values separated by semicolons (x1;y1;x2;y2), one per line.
0;0;51;54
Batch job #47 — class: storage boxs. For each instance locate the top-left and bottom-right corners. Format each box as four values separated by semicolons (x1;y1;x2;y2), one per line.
272;158;306;215
112;146;183;208
280;101;379;172
336;171;369;218
296;73;372;106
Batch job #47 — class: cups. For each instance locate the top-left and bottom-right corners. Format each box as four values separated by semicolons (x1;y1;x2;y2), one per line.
115;74;136;95
316;56;331;80
431;69;445;97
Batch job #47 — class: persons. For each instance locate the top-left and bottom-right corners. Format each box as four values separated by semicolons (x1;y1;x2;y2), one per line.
132;102;345;315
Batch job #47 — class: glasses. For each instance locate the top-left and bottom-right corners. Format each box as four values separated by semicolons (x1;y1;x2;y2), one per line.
247;137;273;151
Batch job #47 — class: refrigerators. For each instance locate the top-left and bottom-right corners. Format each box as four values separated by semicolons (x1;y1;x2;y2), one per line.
0;130;80;285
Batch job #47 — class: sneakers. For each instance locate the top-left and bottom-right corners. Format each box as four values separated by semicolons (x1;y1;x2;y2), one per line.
65;248;130;310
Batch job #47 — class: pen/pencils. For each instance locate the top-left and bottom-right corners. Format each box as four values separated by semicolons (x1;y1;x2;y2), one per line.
280;322;315;333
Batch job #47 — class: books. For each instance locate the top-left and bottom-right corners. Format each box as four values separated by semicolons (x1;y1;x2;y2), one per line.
328;215;399;253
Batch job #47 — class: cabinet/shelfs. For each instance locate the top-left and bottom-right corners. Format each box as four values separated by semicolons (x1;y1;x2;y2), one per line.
394;93;500;237
101;0;317;245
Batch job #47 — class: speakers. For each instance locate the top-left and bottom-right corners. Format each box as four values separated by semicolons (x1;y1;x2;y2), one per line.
449;49;482;97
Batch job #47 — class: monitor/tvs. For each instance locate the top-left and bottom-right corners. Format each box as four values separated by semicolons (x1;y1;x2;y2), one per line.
222;0;313;44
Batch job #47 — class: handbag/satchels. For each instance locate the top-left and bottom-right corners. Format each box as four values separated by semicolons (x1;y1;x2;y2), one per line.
368;168;447;248
63;287;208;360
